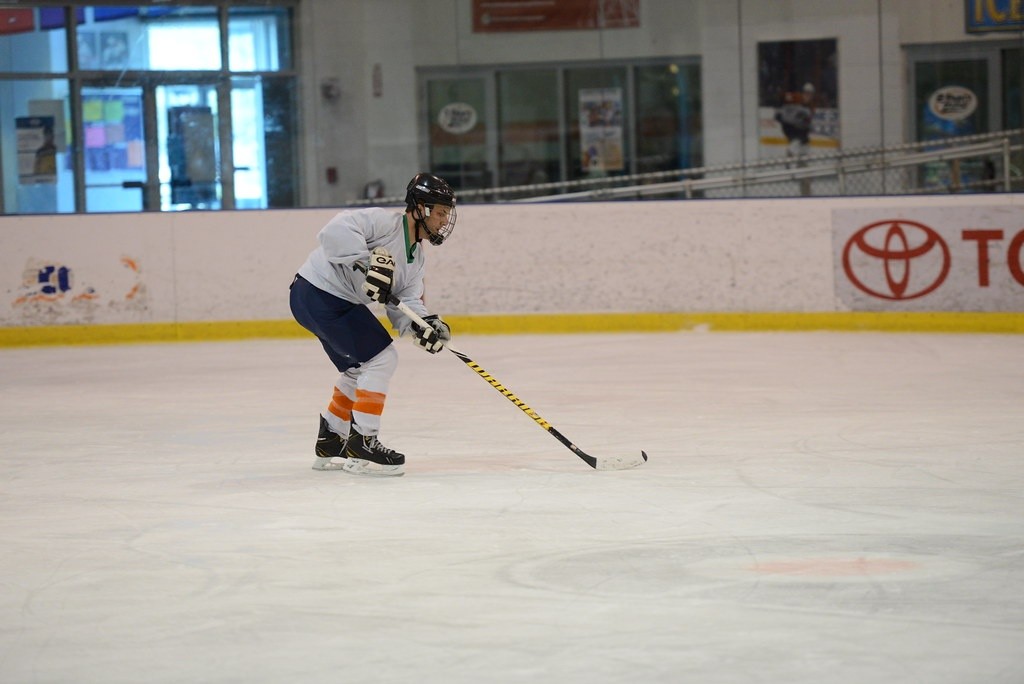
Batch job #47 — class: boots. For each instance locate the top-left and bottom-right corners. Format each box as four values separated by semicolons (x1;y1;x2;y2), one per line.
312;410;406;479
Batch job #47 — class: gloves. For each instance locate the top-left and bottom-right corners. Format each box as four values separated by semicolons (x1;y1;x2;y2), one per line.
361;247;396;305
410;314;452;355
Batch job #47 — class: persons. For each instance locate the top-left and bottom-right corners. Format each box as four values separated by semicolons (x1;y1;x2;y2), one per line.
33;127;57;183
775;80;815;168
290;172;455;475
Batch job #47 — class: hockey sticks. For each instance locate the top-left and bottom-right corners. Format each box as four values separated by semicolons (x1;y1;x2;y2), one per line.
353;260;648;471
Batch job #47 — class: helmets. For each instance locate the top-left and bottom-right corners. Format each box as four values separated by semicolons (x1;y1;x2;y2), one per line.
405;172;457;247
